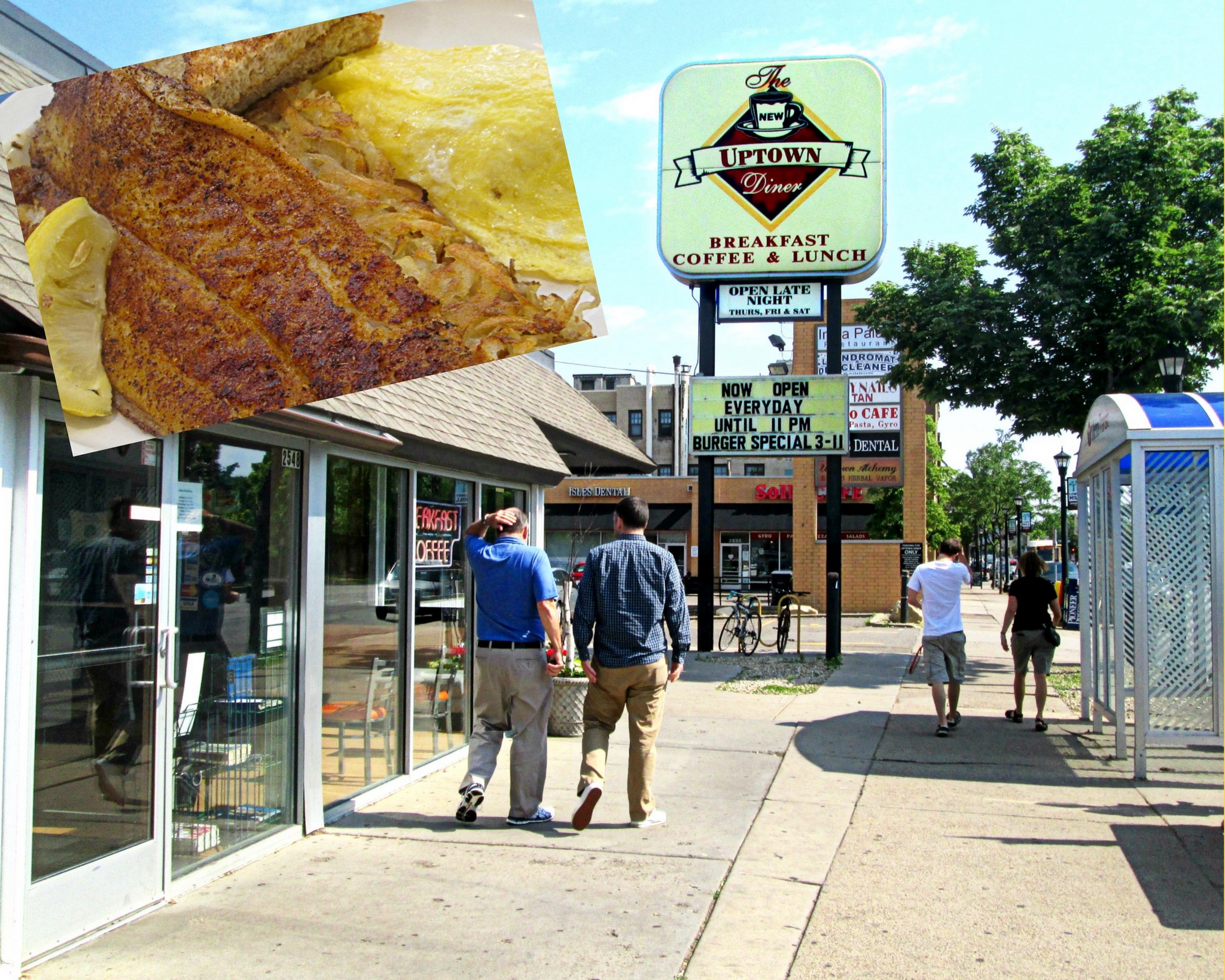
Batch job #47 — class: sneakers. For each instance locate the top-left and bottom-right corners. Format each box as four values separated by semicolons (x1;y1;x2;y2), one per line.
456;783;485;822
95;758;129;808
506;807;554;824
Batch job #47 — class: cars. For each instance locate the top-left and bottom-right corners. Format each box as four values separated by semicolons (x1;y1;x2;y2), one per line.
1044;560;1078;584
375;558;465;623
570;562;585;581
970;554;1017;580
552;568;580;623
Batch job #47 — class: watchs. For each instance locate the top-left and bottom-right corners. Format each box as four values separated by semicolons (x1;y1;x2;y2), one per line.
481;513;489;526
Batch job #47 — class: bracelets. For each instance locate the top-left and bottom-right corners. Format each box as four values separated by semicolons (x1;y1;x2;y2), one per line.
1001;633;1005;634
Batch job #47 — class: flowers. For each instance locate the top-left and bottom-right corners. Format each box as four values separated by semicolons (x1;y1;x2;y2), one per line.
546;645;593;675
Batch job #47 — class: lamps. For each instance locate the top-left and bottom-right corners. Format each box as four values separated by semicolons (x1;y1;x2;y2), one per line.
768;363;789;376
768;334;785;351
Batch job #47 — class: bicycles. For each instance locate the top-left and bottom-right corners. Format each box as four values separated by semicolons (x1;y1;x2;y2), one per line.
768;587;811;654
718;590;762;656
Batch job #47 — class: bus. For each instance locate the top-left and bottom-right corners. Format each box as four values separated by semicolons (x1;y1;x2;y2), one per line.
1027;540;1062;562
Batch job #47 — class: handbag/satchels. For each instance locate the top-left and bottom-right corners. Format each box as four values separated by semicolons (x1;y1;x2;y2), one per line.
1046;626;1060;648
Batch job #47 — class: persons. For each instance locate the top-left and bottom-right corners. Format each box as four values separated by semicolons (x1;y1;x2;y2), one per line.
1000;551;1063;732
451;507;565;824
571;495;692;831
906;539;974;737
58;497;233;809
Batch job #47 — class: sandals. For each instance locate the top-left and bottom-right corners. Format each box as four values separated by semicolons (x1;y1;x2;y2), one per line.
935;725;949;738
946;711;961;728
1005;709;1023;723
1035;719;1048;731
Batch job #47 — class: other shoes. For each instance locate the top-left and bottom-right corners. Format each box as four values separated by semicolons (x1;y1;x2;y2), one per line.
630;807;666;827
571;784;602;831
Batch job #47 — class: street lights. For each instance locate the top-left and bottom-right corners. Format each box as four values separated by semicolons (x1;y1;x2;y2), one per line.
1002;506;1012;593
1013;494;1024;579
1053;446;1073;612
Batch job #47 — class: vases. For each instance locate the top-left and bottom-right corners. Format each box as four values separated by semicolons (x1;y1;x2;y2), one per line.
551;676;589;738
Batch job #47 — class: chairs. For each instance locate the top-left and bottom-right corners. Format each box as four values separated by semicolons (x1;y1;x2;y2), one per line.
324;652;394;785
414;642;458;755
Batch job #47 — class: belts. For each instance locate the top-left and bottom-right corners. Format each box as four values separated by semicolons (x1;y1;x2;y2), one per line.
478;640;543;649
